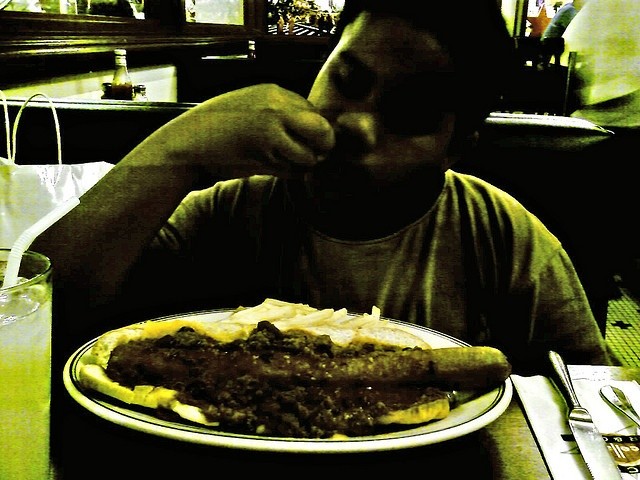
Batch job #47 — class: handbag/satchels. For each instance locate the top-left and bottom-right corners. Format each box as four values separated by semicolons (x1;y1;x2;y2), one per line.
0;90;116;249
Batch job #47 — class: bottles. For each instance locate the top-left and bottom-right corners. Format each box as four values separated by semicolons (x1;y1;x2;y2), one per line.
110;47;131;98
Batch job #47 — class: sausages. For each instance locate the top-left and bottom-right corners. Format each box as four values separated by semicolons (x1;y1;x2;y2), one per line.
108;341;511;391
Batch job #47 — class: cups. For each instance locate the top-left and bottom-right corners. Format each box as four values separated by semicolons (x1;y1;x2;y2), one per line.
0;249;52;480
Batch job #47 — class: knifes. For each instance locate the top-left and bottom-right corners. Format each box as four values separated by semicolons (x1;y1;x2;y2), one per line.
542;346;625;475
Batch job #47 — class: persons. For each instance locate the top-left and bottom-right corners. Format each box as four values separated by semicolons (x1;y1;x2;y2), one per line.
29;0;611;375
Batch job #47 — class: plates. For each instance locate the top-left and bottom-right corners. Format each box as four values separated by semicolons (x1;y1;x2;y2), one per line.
62;309;516;453
